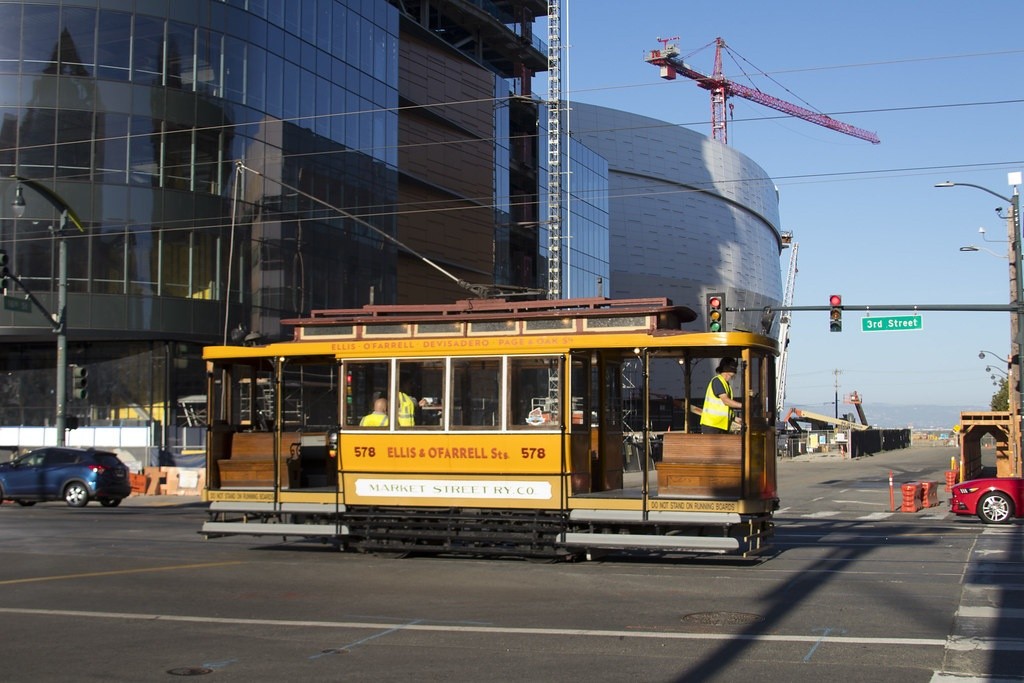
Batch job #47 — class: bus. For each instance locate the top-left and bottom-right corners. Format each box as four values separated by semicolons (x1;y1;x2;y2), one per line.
200;284;780;561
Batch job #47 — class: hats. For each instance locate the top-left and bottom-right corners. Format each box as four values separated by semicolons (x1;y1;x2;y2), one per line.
716;358;737;374
373;391;386;401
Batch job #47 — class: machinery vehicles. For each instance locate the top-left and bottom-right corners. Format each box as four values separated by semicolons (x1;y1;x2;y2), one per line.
774;241;811;435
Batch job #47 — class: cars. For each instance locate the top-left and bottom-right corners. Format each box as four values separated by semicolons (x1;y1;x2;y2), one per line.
0;448;131;508
952;477;1024;525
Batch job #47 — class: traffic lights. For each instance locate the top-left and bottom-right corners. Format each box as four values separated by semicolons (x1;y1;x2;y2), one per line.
829;294;843;331
0;247;10;292
707;292;726;331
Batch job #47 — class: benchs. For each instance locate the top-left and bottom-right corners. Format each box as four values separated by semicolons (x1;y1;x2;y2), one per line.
215;432;301;490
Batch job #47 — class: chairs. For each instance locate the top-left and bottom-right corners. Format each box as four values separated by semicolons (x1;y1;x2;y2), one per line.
422;394;582;427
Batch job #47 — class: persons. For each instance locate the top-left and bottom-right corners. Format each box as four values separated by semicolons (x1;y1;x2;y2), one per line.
360;397;388;426
25;387;50;426
700;356;743;434
399;373;427;428
9;447;18;461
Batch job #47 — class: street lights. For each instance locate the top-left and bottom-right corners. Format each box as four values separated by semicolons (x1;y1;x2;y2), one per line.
0;174;84;448
832;367;843;418
933;171;1024;472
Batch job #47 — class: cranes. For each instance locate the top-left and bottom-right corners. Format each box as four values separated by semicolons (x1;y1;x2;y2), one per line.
642;35;883;146
